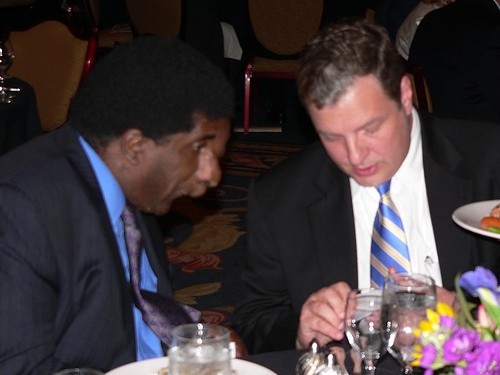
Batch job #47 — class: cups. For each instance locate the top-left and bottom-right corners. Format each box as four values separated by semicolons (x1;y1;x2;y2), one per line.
167;324;233;375
54;368;105;375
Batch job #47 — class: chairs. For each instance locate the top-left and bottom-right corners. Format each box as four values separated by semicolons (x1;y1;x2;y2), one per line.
0;0;328;134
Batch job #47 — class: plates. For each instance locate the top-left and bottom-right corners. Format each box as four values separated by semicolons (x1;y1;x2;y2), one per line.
451;200;500;239
105;356;278;375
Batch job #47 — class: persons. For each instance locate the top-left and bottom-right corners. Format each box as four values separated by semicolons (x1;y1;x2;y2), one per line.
235;17;500;355
1;35;237;375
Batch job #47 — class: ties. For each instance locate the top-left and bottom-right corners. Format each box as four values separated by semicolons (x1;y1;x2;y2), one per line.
370;180;413;292
121;205;202;348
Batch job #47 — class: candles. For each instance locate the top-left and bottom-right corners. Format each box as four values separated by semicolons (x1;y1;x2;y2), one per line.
245;64;252;133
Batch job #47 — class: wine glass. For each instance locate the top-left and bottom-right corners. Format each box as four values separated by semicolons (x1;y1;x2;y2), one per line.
380;272;438;375
345;288;396;375
0;41;16;103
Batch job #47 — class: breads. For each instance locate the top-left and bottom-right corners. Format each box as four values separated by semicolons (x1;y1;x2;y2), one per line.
481;217;500;230
